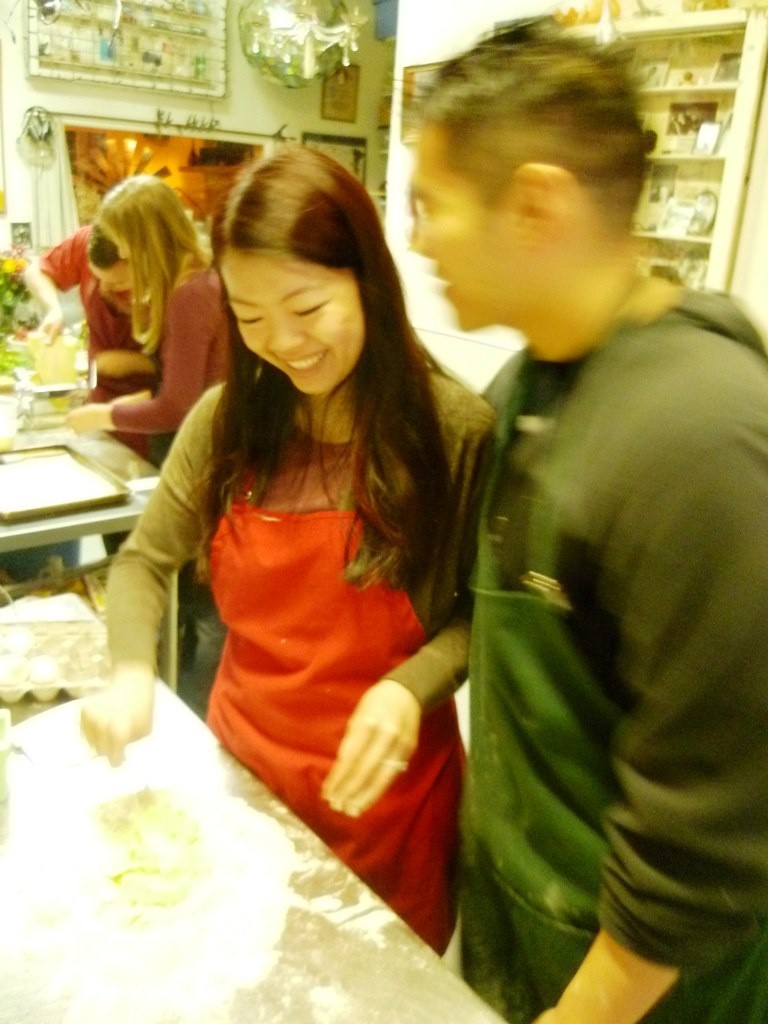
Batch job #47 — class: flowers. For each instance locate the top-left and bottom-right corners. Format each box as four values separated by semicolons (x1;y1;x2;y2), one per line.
0;253;30;317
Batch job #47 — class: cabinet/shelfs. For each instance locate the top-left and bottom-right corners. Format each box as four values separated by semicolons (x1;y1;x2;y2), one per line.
561;5;768;295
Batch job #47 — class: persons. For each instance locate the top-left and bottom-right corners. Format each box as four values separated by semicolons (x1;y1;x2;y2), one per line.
78;142;495;957
37;225;150;460
404;36;767;1024
66;173;232;668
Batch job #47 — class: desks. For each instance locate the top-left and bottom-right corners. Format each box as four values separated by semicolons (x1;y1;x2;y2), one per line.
0;676;508;1024
1;393;178;695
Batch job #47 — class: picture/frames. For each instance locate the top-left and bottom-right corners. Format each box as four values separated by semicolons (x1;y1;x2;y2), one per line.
400;58;453;144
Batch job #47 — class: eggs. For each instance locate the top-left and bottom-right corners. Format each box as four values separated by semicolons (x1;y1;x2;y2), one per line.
0;629;60;687
